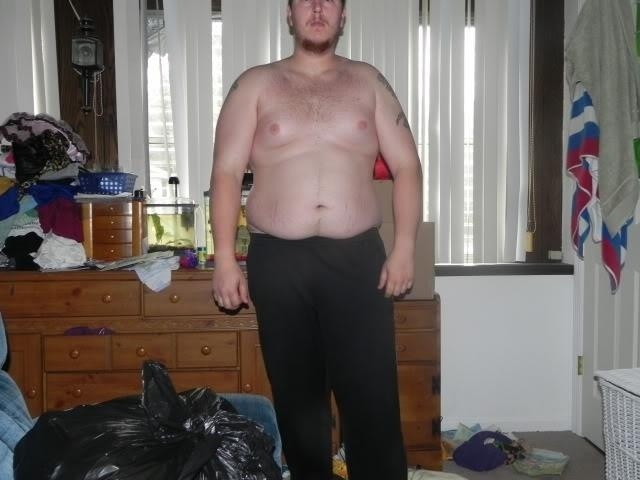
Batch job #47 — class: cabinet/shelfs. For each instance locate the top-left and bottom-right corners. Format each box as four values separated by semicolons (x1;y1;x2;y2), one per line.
393;294;442;470
0;270;274;418
80;199;143;261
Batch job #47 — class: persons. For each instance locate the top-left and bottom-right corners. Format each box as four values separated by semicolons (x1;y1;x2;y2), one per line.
213;0;422;480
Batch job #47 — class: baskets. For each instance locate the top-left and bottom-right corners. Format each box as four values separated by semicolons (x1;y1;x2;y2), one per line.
77;173;138;196
598;377;640;480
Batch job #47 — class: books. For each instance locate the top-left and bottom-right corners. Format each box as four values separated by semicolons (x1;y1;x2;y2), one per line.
96;249;175;274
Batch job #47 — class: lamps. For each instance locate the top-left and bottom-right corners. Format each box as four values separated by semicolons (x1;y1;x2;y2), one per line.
69;16;104;117
